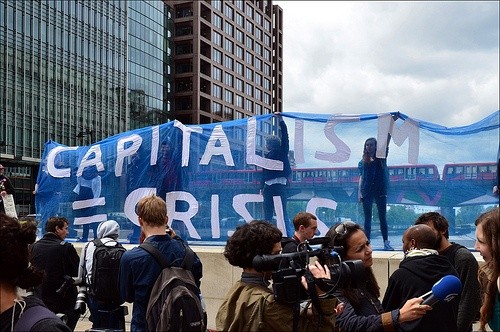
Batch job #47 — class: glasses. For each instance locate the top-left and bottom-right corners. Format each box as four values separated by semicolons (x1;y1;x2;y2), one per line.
335;220;356;236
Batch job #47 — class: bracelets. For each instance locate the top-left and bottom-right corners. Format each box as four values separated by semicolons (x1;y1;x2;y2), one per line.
391;309;401;325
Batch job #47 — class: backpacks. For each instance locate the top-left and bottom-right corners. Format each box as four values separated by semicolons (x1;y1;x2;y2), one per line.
87;238;127;307
139;242;207;332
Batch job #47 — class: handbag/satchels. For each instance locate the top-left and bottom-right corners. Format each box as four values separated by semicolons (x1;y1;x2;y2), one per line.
56;280;87;316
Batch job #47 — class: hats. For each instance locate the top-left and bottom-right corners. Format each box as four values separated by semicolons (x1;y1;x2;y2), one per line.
0;164;5;169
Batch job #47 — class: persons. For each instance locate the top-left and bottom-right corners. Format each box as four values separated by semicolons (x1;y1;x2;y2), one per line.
33;154;62;235
357;113;399;252
71;166;101;243
213;208;500;332
117;128;203;332
0;165;16;214
491;158;500;205
0;211;130;332
259;112;294;238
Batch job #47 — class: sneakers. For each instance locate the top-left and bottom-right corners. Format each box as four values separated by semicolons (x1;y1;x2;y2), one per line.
384;240;394;250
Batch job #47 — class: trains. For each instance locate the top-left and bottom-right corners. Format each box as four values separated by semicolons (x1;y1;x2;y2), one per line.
183;160;499;189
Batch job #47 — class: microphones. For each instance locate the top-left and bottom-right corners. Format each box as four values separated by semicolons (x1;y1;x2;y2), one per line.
405;276;462;325
301;236;331;245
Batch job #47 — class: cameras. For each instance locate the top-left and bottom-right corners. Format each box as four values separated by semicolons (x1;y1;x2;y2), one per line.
73;279;92;315
56;275;74;297
